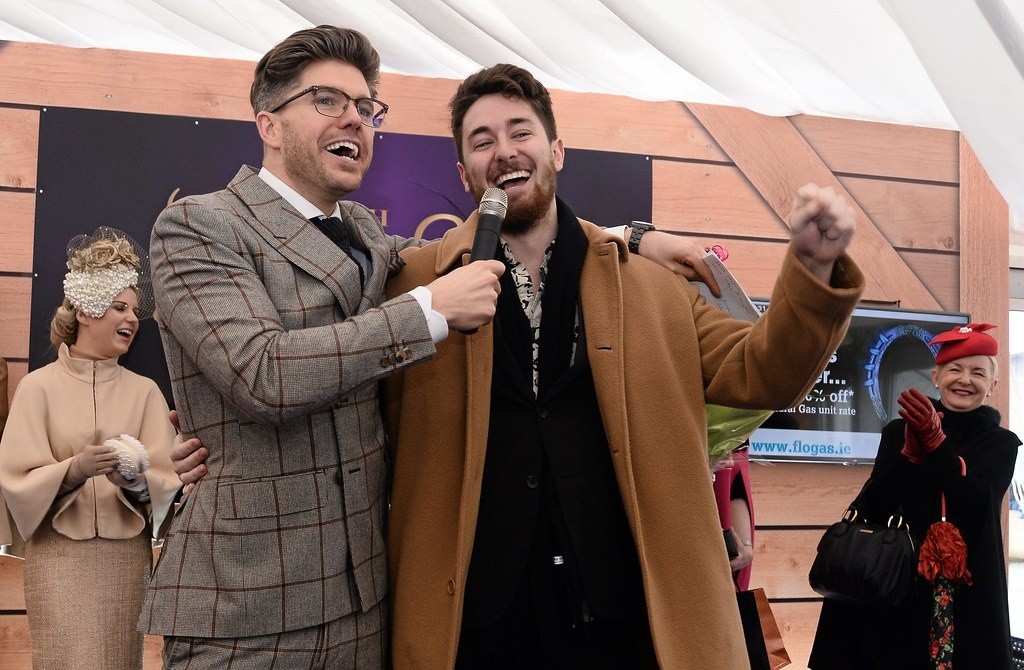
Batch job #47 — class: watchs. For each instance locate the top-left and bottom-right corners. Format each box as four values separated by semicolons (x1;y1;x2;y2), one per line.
628;220;655;255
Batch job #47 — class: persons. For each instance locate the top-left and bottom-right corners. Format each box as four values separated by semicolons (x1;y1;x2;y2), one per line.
0;226;180;670
809;323;1023;670
135;24;722;670
712;437;755;591
168;63;865;670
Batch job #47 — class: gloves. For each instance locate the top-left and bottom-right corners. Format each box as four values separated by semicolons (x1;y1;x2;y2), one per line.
900;412;943;464
898;387;946;454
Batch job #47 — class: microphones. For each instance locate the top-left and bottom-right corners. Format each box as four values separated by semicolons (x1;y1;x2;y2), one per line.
457;189;507;334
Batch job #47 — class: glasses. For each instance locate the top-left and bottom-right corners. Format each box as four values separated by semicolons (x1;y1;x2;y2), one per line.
268;85;390;129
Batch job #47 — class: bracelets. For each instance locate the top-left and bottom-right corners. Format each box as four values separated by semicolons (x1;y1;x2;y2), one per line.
742;540;752;546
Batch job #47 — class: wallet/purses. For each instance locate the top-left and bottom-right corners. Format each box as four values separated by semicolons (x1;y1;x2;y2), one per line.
724;530;739;558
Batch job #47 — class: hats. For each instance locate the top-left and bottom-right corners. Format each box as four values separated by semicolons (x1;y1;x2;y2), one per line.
930;323;999;365
63;233;141;319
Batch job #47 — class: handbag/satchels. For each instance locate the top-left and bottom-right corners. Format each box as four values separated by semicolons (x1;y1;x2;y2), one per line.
734;588;792;670
809;505;916;606
